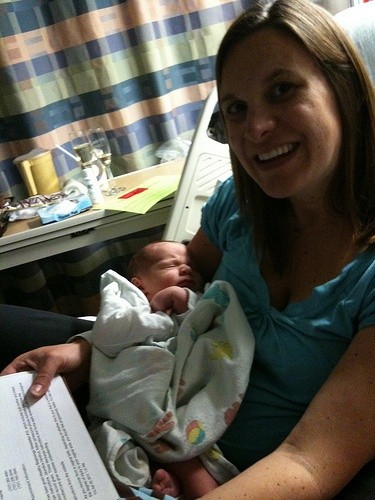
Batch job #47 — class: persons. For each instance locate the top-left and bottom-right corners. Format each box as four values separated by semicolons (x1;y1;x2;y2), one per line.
123;235;221;500
0;0;375;500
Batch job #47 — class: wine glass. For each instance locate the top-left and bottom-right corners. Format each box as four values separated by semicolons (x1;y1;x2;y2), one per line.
70;131;109;196
87;127;125;195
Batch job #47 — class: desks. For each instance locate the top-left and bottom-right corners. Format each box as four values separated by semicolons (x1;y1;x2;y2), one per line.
0;159;184;288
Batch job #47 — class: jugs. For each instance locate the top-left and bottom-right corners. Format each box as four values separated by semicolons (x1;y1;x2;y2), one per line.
13;149;60;198
